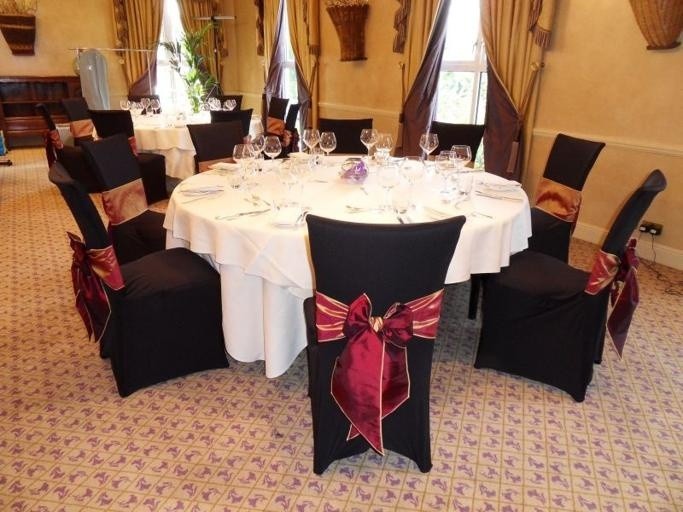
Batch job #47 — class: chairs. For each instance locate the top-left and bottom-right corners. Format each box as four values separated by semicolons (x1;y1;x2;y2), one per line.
82;131;166;264
187;120;244;173
428;122;486;163
86;109;168;204
62;98;98;146
320;117;373;156
219;95;242;110
127;94;160;115
211;109;253;143
266;97;303;158
301;214;467;476
522;133;606;262
49;161;229;399
37;104;104;193
474;169;667;403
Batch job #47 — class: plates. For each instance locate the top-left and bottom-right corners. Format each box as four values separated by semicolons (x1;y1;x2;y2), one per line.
479;178;521;192
172;120;189;128
211;160;238;172
263;206;305;228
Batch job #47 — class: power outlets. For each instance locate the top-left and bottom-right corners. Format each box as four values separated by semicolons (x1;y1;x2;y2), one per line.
642;220;663;236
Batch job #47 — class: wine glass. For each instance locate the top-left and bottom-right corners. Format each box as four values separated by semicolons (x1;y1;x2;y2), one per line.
122;100;132;110
234;145;254;187
142;98;151;114
294;159;311;206
208;98;226;111
265;137;281;163
418;133;439;162
373;133;393;161
151;99;159;115
226;100;236;111
320;133;337;160
441;150;455;192
304;129;319;154
451;142;470;172
248;132;265;160
390;185;411;223
360;127;375;158
278;155;297;205
133;102;142;115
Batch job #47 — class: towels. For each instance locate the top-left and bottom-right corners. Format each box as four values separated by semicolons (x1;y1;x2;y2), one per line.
181;186;223;198
276;205;305;228
